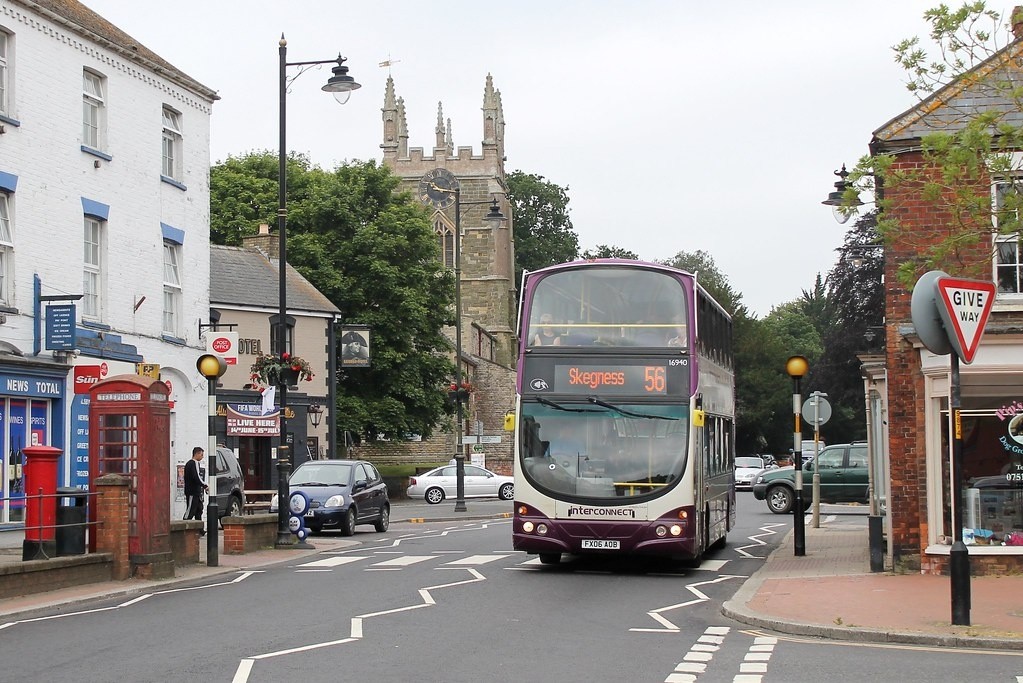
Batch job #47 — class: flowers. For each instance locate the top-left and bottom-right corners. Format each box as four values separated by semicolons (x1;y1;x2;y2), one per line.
249;350;316;394
450;380;475;392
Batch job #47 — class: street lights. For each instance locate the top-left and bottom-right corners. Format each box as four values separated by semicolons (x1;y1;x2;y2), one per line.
455;188;509;512
786;356;808;556
196;354;227;566
274;32;362;549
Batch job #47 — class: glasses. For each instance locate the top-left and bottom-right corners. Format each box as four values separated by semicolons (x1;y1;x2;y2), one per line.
541;321;552;325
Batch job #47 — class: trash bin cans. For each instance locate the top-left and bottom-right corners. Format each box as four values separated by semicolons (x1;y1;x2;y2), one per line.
55;487;87;556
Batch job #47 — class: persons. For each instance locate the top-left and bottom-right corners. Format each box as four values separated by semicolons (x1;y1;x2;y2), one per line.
535;313;561;346
544;423;585;457
182;447;208;537
668;327;687;347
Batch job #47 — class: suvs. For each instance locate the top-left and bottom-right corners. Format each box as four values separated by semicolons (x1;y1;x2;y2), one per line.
751;440;870;514
789;439;825;464
216;442;247;529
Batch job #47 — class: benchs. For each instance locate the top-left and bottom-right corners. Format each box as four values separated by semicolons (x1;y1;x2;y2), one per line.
242;501;271;515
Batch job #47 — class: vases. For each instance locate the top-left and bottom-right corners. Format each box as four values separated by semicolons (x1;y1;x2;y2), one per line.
268;367;300;386
449;392;470;402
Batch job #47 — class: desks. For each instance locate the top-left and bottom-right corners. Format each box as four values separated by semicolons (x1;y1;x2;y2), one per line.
244;489;278;501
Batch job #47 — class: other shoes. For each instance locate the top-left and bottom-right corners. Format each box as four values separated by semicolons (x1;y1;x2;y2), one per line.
200;530;206;536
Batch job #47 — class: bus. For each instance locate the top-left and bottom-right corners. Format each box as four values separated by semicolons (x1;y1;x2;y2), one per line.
503;256;736;568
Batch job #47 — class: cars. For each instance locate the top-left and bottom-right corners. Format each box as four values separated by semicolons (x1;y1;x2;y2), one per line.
406;464;514;503
269;458;390;537
756;454;793;471
734;456;768;491
